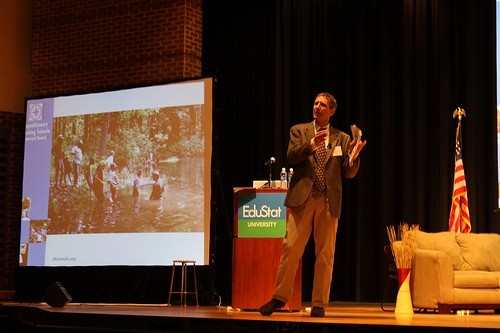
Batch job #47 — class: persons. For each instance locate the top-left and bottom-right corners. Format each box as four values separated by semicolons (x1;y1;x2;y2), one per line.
52;134;82;190
260;93;360;317
83;150;120;204
149;170;164;200
133;169;141;197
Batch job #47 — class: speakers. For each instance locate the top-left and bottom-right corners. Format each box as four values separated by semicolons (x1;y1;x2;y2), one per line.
46;281;71;308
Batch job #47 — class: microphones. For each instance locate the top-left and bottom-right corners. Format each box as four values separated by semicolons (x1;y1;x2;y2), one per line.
269;157;276;164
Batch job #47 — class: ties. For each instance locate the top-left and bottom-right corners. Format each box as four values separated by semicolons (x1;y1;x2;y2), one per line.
315;128;328;192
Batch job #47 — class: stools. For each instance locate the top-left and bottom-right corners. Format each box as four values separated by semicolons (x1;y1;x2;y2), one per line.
166;260;198;308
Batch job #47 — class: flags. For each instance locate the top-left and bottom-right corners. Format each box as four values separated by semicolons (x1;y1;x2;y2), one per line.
448;116;471;233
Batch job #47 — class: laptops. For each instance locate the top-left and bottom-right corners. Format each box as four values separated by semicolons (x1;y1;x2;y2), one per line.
253;180;288;189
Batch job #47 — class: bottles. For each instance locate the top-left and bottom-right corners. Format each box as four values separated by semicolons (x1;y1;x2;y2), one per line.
288;168;293;188
280;168;287;190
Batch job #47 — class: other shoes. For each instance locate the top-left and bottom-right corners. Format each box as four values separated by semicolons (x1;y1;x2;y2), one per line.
260;299;285;316
311;306;325;317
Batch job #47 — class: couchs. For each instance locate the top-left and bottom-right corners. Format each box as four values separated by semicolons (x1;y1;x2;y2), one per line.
410;232;500;313
